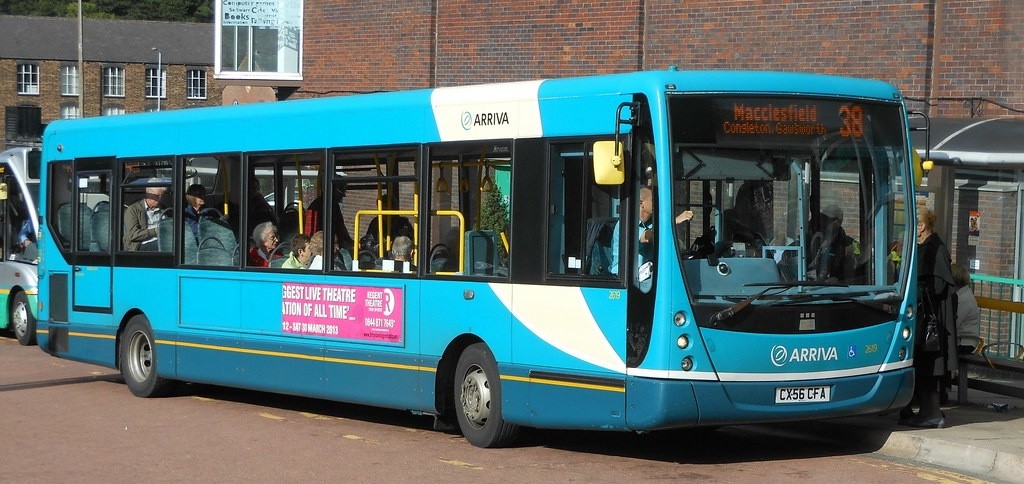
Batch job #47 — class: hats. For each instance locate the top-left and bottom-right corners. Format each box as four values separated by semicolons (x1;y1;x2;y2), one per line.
820;204;843;220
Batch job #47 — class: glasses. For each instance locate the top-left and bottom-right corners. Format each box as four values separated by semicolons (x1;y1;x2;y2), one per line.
269;234;280;240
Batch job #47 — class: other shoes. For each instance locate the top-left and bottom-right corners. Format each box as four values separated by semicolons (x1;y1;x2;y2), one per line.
897;415;944;429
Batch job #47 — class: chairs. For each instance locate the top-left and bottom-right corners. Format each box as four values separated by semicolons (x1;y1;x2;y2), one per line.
587;216;619;275
54;199;456;275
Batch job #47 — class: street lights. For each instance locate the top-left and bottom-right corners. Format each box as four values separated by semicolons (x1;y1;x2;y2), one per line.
150;46;163;113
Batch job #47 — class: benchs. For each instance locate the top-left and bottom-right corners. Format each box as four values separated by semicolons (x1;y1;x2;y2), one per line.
953;352;1024;405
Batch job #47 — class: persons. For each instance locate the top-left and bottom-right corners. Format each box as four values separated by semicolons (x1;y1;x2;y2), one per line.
122;172;417;271
887;206;980;428
15;217;34;253
608;185;694;294
724;182;895;287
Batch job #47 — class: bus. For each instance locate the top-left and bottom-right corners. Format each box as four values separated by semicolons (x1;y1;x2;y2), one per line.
82;165;344;221
35;66;937;448
0;143;195;347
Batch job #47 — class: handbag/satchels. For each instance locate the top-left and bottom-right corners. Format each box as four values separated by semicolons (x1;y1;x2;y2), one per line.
920;287;941;351
